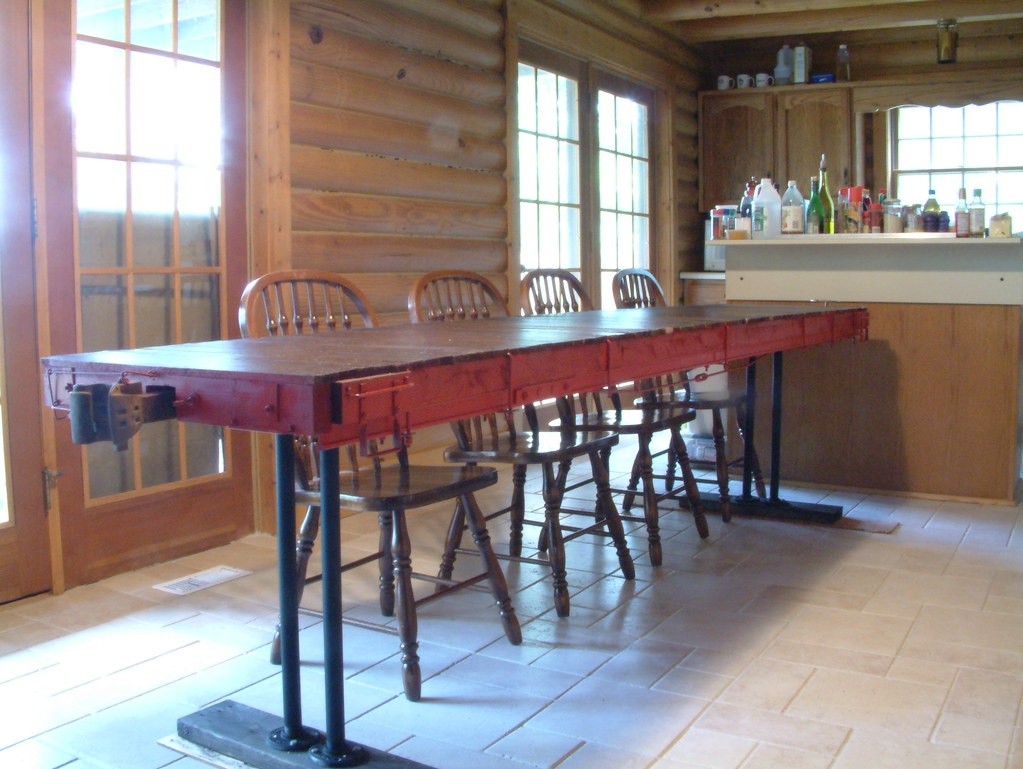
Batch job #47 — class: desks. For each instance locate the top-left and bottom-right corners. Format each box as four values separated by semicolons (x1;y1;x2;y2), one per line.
40;301;870;769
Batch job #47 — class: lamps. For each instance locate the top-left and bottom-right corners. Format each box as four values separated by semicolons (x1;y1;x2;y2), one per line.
936;17;959;66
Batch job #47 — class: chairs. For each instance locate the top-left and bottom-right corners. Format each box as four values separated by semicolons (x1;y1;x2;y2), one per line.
238;268;767;703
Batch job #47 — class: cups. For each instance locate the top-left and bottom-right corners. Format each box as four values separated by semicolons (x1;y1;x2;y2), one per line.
717;75;735;90
737;74;755;89
756;73;774;87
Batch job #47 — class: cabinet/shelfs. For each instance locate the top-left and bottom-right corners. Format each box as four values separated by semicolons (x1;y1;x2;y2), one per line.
678;272;725;306
698;83;864;216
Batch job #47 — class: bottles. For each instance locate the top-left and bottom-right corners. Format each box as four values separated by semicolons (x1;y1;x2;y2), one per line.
773;45;794;85
710;161;986;238
835;44;851;82
